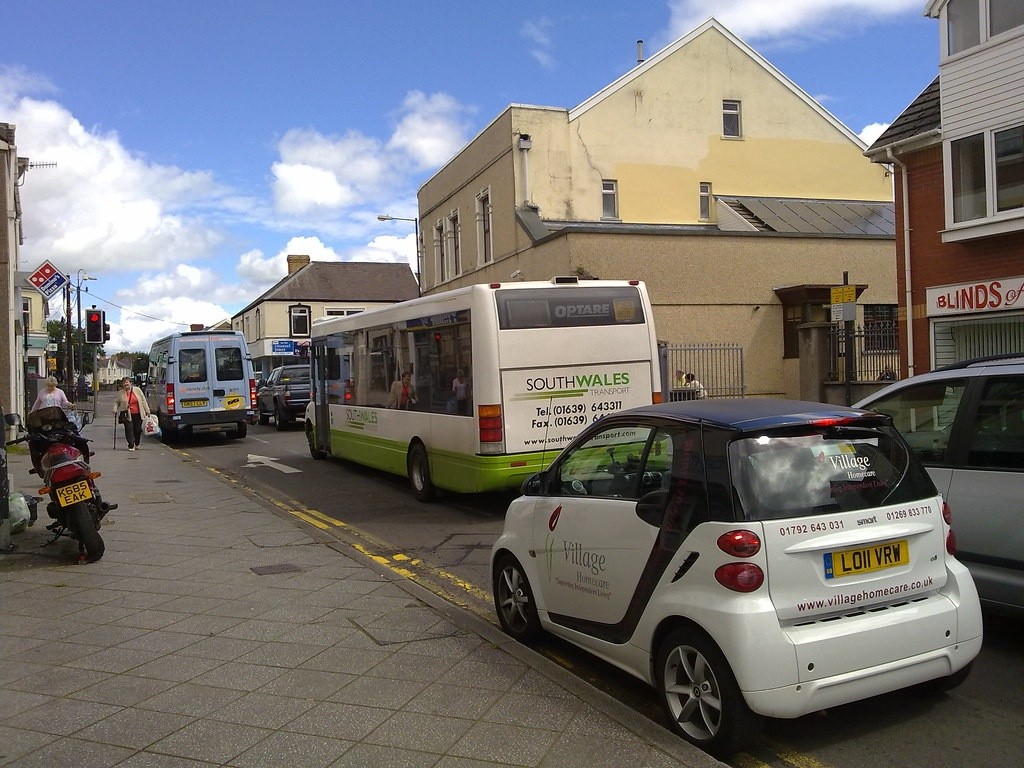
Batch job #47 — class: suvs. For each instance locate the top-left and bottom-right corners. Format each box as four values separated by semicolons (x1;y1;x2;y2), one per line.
255;365;311;432
802;352;1024;620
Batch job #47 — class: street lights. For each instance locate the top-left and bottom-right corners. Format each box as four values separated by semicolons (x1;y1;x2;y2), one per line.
377;214;422;297
76;268;88;402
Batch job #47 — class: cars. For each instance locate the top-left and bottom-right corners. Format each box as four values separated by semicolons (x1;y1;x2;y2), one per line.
489;394;983;755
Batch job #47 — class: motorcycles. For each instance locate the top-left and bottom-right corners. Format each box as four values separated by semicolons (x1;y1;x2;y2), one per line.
4;406;119;563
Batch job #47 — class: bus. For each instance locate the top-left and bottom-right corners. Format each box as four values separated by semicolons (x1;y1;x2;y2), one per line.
299;272;663;503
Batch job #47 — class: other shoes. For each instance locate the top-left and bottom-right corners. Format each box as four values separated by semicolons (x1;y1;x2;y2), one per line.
135;443;139;449
129;446;135;450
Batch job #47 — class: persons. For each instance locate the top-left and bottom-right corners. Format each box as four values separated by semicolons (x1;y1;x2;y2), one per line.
113;377;151;452
686;373;708;401
674;369;687;388
877;365;897;382
385;372;417;410
451;367;473;416
27;377;76;415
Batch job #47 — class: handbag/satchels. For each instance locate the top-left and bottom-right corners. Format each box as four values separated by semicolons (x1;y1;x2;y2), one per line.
446;391;458;413
408;395;416;404
144;415;159;435
142;414;158;430
66;404;81;430
118;409;132;424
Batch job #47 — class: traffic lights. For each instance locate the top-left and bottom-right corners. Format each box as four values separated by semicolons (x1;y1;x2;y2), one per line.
102;309;111;343
84;308;105;345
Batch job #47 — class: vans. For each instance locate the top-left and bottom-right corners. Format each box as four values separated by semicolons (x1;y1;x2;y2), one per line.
137;331;258;444
